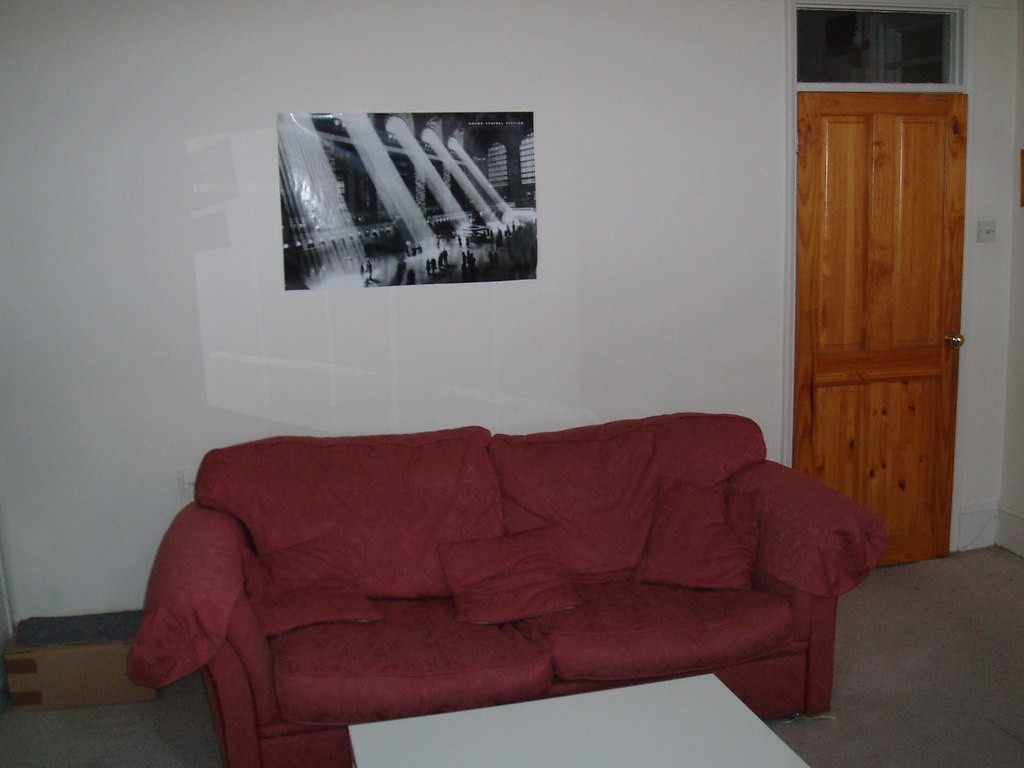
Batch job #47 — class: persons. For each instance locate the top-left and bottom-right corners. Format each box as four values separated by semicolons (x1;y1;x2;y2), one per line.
357;220;537;286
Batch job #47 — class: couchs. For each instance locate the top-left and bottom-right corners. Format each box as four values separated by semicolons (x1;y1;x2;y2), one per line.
126;412;889;768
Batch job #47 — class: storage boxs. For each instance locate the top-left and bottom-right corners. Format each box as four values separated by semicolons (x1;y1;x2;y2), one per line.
4;623;157;713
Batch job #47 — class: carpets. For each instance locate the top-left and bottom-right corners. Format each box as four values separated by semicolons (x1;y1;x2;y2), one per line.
17;610;142;646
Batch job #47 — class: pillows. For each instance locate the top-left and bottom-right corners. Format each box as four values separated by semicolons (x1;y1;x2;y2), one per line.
437;524;585;625
241;530;380;638
633;477;766;591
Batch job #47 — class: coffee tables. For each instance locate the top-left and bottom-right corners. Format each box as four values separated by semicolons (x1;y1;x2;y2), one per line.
347;673;814;768
